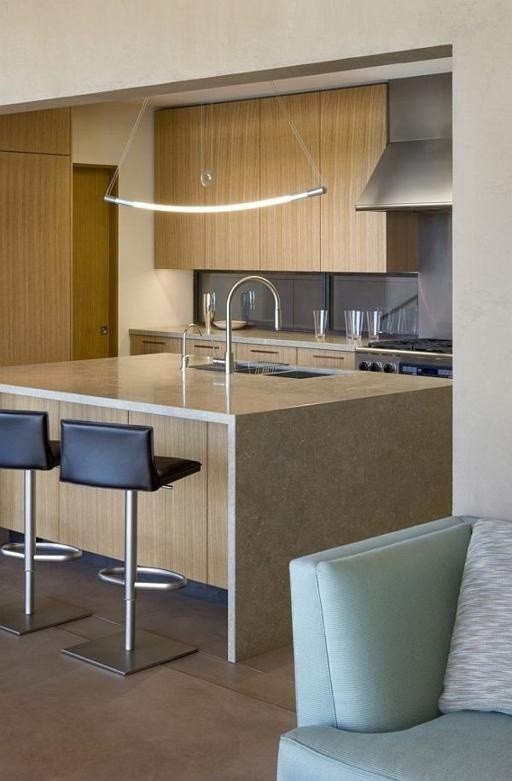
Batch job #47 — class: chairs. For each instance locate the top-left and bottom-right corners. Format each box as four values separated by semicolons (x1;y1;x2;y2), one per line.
0;411;94;637
58;421;206;675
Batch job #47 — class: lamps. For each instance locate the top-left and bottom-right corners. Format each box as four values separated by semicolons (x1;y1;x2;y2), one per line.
102;79;330;213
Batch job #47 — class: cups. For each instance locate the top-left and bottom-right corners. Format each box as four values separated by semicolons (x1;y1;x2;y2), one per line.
343;310;380;343
313;310;329;337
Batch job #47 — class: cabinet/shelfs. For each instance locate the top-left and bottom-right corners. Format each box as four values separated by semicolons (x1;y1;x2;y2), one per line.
0;107;72;366
129;338;355;370
153;83;422;274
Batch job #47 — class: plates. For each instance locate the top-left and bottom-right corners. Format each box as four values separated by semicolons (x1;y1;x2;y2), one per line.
212;321;246;330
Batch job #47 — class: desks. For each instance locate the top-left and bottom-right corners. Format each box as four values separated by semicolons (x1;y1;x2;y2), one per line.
0;352;453;664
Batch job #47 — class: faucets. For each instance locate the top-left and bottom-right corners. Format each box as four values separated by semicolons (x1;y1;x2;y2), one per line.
182;323;203;357
226;275;282;352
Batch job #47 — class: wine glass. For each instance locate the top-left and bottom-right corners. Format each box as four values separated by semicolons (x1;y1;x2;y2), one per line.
240;292;255;329
202;292;214;336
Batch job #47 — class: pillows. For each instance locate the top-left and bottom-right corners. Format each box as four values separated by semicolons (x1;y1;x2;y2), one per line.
436;519;512;715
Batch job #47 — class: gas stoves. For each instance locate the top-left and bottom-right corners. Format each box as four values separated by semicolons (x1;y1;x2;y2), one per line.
369;338;451;355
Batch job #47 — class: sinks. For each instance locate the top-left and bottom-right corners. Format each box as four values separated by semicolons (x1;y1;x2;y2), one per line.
206;364;256;370
264;371;333;380
235;368;289;375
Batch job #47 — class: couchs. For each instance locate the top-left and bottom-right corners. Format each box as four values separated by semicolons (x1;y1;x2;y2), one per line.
276;515;512;781
